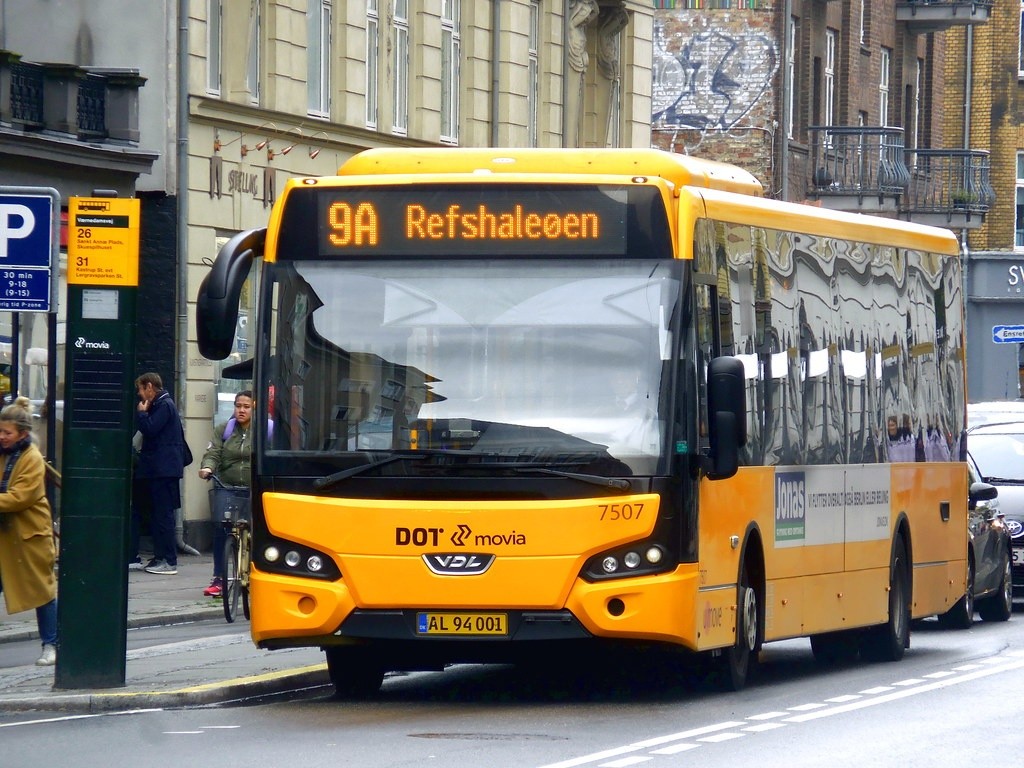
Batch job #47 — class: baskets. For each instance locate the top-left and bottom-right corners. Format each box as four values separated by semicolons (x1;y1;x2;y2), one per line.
208;488;250;526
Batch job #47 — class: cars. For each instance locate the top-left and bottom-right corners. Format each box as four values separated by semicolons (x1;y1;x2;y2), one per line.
912;449;1015;629
966;420;1024;613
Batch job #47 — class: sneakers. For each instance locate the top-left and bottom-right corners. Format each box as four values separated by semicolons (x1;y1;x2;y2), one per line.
144;558;178;574
203;578;224;596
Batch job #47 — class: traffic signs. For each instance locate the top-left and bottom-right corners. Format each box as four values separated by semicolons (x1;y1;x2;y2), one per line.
991;324;1024;345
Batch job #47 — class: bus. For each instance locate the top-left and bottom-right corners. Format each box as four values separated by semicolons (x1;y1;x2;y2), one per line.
194;143;971;704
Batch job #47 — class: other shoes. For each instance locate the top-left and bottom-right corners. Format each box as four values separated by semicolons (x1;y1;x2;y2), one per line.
35;643;56;665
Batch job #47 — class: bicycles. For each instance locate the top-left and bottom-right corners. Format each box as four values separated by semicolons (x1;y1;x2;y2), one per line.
207;471;253;624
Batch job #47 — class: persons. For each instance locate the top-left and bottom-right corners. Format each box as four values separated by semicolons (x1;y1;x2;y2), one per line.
129;372;185;575
198;389;254;597
0;395;58;667
888;416;910;441
